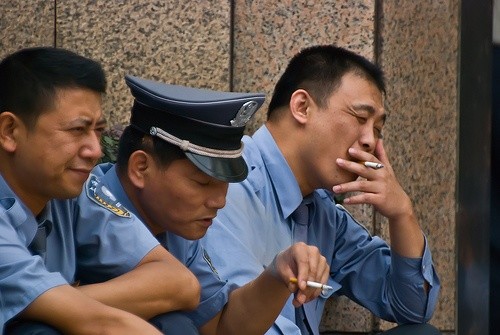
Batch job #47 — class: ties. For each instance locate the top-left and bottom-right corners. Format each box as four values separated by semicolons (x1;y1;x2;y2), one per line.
292;205;307;249
32;225;48;265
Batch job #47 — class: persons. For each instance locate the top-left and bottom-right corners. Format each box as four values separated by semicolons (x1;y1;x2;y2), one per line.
91;72;332;335
0;46;201;335
200;44;444;335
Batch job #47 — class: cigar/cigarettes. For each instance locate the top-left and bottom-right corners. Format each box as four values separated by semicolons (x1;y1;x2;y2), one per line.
359;160;385;171
287;273;334;291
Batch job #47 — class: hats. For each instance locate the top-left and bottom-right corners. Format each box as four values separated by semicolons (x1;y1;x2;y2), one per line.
125;73;266;183
